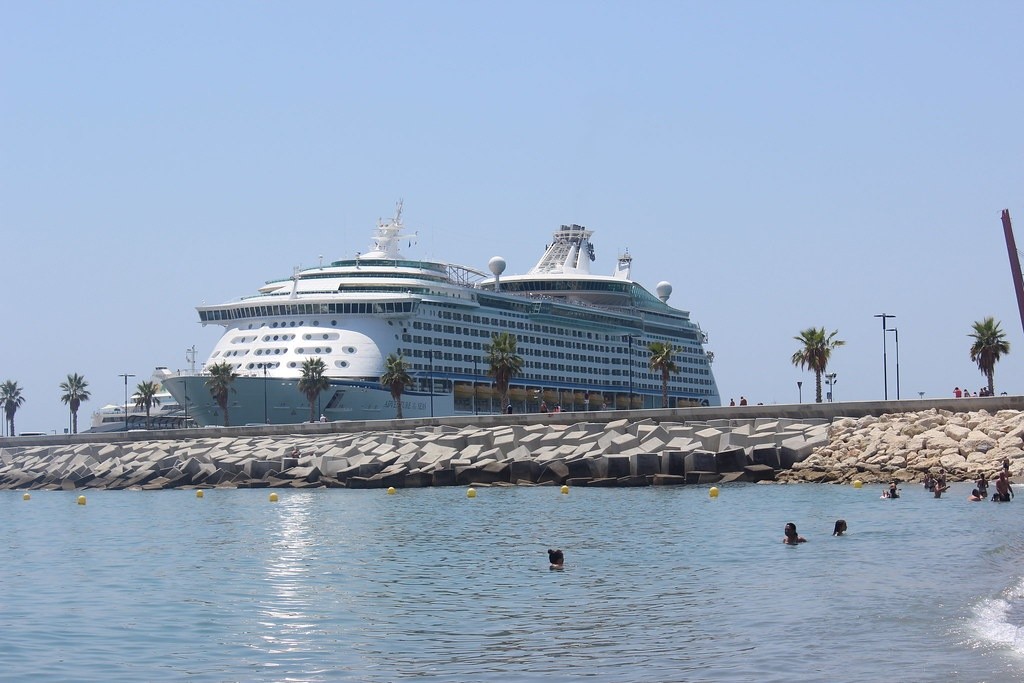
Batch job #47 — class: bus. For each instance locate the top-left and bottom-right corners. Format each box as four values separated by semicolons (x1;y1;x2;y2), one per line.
18;431;47;436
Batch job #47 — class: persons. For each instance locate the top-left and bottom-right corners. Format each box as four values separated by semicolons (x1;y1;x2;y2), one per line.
602;402;607;410
970;392;978;397
977;474;989;498
552;404;560;412
963;389;970;397
548;549;564;569
783;523;807;544
540;400;547;412
953;387;961;397
924;469;950;498
979;387;994;396
883;478;900;498
833;519;847;536
969;489;981;501
320;413;326;423
506;403;512;414
730;398;735;406
1003;456;1009;473
740;396;747;406
991;472;1014;502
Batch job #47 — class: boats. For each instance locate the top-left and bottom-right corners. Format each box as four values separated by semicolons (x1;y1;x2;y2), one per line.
90;345;205;433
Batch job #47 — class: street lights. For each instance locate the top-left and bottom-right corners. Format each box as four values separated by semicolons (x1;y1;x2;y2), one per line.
118;373;136;431
51;429;57;435
872;312;897;400
470;359;479;415
621;332;641;409
917;391;926;399
797;381;803;403
886;327;900;400
428;348;442;416
824;372;837;402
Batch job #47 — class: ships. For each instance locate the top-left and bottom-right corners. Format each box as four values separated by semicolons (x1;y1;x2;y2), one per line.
157;192;722;429
